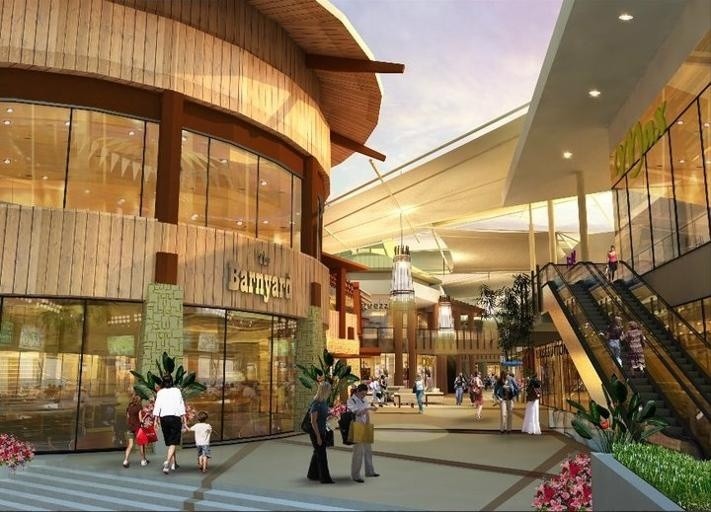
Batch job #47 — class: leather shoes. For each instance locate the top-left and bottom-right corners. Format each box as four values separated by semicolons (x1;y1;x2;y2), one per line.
162;467;169;475
172;464;179;470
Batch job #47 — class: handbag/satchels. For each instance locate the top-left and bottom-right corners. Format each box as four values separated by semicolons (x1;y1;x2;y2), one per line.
135;424;158;445
471;382;481;396
347;415;375;445
338;411;358;446
413;384;417;393
325;425;334;447
300;411;313;436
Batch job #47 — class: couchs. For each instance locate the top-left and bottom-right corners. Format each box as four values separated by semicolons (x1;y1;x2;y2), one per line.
365;384;444;408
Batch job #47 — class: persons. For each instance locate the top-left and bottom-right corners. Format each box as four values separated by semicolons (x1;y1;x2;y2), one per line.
452;368;542;434
599;316;624;368
185;411;213;473
346;383;379;483
306;381;336;484
607;245;619;283
369;375;390;407
153;374;187;474
413;373;426;413
622;321;646;377
121;395;150;466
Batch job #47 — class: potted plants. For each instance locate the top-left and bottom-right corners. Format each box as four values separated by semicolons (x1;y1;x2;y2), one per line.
295;348;359;448
131;354;207;456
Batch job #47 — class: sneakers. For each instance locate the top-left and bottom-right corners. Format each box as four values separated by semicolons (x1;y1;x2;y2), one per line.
122;460;130;468
140;458;150;466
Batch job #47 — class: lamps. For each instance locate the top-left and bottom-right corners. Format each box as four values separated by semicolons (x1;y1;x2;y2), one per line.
435;258;456;336
388;211;418;304
